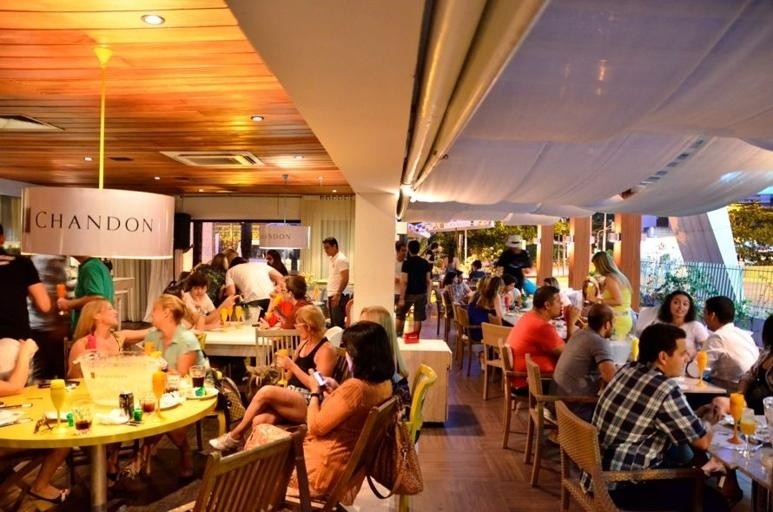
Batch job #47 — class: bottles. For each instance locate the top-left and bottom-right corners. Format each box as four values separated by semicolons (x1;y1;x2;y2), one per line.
504;286;534;314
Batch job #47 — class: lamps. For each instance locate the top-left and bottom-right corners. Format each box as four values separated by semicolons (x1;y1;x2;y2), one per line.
258;196;310;249
19;47;176;260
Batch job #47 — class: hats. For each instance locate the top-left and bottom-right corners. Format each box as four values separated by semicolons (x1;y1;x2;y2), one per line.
505;235;523;248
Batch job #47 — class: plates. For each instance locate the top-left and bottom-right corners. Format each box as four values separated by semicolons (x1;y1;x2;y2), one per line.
45;412;67;420
186;387;219;400
0;412;23;426
158;396;183;410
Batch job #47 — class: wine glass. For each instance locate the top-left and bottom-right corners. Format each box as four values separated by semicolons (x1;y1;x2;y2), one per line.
54;282;67;316
150;373;167;419
219;304;260;328
728;392;773;472
276;349;289;385
48;379;66;433
583;282;593;304
265;293;281;317
695;352;707;388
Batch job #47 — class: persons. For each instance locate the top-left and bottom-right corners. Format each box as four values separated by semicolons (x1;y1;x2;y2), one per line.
506;251;772;511
0;230;414;510
389;237;539;373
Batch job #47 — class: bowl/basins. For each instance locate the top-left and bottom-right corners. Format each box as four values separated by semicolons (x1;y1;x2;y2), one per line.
78;349;168;406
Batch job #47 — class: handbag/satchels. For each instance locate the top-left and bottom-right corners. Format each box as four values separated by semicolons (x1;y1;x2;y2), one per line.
212;376;245;422
396;383;410;419
371;421;423;495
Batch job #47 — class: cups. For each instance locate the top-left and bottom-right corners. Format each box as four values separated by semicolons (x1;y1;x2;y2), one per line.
132;408;142;421
65;413;72;426
144;341;155;354
138;399;155;412
631;337;639;362
188;365;205;386
71;405;92;434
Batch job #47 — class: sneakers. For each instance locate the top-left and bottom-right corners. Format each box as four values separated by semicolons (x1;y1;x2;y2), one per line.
209;433;241;451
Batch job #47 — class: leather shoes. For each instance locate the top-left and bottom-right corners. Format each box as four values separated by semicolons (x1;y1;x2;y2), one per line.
180;453;193;476
108;472;118;481
124;452;145;477
28;485;66;503
